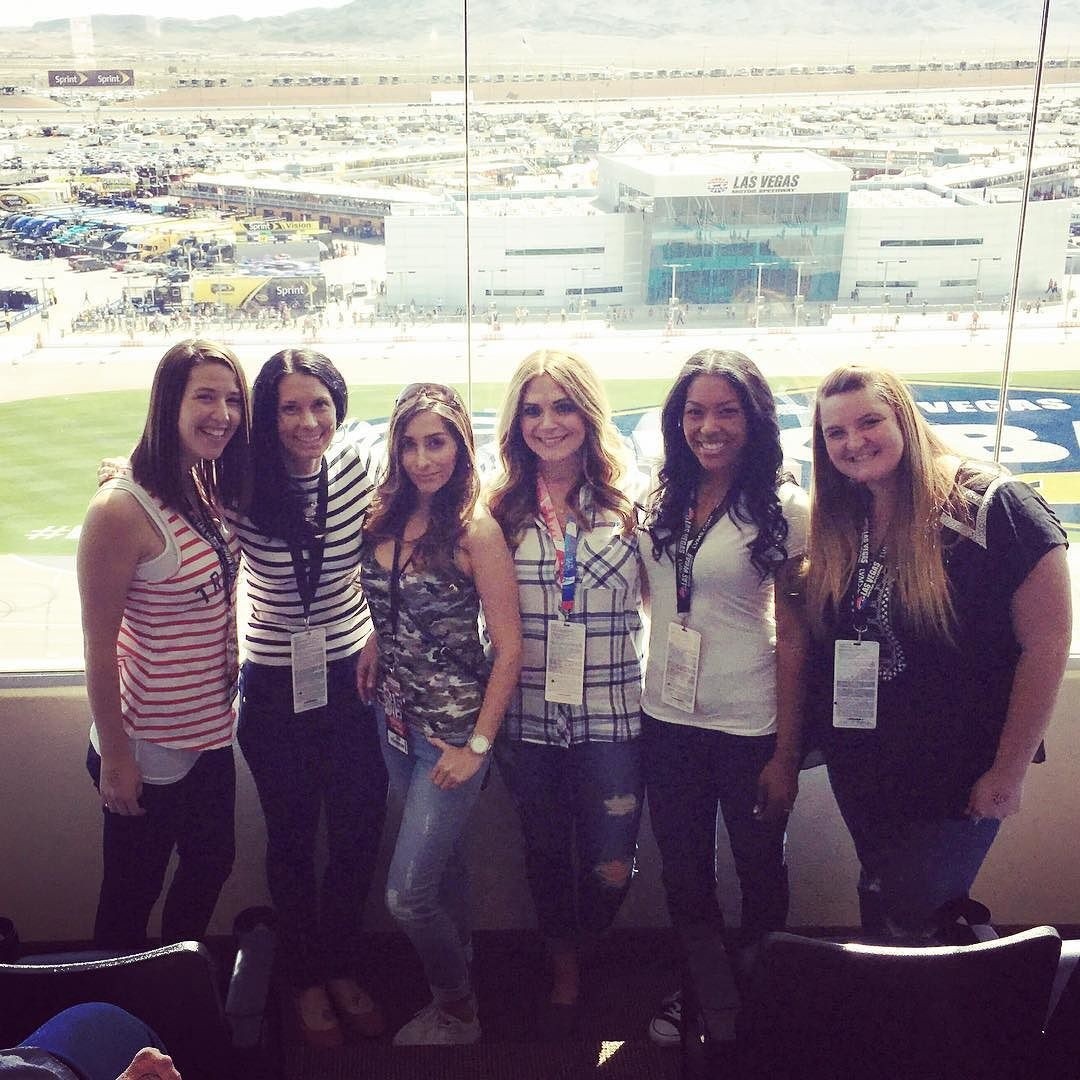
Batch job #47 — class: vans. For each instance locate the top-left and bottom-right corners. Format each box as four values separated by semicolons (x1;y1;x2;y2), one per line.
0;286;57;310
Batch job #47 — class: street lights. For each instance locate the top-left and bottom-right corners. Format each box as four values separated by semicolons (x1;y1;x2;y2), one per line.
573;258;1001;313
387;271;415;303
478;270;506;321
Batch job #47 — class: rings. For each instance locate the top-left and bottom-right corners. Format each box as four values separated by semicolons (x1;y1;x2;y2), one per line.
783;809;793;813
106;803;116;809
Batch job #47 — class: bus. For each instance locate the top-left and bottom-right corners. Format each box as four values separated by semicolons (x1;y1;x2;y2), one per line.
77;259;97;272
69;256;90;266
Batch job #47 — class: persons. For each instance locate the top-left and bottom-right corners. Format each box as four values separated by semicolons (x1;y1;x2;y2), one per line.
0;1002;183;1080
802;364;1075;943
77;339;253;1031
631;351;810;1048
481;350;649;1024
356;383;524;1045
236;348;392;1053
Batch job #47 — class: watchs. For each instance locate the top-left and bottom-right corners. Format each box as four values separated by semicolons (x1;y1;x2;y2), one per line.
465;732;492;756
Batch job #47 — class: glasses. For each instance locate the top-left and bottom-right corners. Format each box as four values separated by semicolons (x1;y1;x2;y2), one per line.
394;383;464;415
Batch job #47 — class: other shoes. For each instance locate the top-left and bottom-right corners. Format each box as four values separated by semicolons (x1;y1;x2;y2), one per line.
293;992;344;1052
330;983;386;1037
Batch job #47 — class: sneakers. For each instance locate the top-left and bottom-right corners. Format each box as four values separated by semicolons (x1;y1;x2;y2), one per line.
648;991;682;1049
391;1000;483;1047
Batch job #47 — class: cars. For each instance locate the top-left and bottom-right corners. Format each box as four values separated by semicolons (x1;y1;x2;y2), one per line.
123;261;149;273
871;60;1080;73
143;263;166;275
719;97;1080;135
177;74;544;89
266;114;461;148
159;266;190;282
113;259;130;271
552;70;706;82
709;64;855;78
470;110;720;143
34;116;249;146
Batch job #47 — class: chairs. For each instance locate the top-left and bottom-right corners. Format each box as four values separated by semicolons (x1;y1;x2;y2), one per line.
0;916;283;1080
672;915;1080;1080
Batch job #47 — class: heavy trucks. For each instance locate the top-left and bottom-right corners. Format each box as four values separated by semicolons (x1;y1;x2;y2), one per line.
160;271;328;317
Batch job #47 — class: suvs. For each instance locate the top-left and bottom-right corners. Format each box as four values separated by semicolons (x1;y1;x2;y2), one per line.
352;280;367;296
